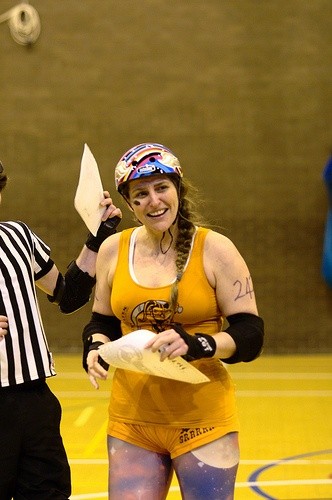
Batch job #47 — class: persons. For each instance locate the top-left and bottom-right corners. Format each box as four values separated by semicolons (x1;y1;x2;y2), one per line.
82;143;264;500
0;162;122;500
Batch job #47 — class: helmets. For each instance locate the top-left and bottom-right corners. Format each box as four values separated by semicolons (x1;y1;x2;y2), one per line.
114;142;184;192
